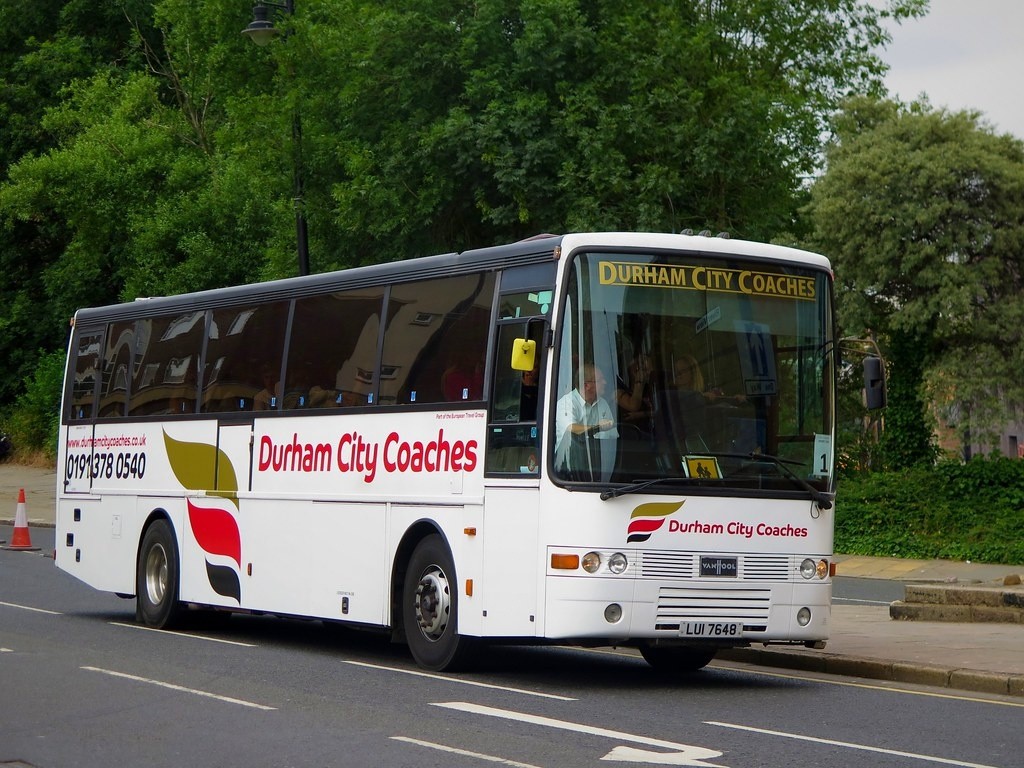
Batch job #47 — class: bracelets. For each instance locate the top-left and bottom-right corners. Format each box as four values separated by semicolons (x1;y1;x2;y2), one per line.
633;380;644;384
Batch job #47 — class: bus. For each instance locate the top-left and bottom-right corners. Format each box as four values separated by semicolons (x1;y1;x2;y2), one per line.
53;230;888;675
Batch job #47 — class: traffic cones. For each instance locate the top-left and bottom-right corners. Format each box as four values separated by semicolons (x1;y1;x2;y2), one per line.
0;488;45;550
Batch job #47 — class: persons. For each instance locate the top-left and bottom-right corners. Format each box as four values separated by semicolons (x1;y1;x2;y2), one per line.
553;364;619;453
215;355;343;411
510;352;540;422
441;340;483;402
617;355;746;438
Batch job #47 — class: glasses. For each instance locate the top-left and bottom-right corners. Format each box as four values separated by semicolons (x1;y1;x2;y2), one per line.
583;376;604;385
